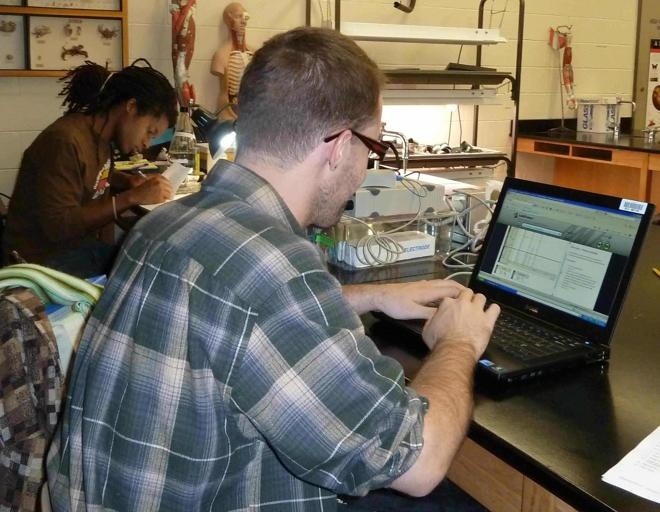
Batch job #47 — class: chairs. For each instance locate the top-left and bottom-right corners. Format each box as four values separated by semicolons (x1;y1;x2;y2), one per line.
0;273;167;512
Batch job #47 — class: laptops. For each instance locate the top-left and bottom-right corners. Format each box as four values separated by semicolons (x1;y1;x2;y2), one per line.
392;177;656;405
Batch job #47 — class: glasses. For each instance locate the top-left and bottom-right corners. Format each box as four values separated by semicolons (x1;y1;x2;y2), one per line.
324;128;391;160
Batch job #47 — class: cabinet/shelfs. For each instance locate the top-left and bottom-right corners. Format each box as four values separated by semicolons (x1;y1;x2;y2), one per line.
304;0;526;180
0;0;130;77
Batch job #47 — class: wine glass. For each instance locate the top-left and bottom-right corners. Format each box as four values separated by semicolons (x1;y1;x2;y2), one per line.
168;131;197;190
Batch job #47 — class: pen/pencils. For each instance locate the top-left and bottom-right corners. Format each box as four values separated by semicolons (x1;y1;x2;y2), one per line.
137;170;149;181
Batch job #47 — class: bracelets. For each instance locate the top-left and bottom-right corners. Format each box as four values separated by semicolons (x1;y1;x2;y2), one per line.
110;194;120;223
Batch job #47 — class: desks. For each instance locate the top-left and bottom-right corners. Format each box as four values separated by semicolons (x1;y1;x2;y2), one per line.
517;131;659;217
109;169;659;512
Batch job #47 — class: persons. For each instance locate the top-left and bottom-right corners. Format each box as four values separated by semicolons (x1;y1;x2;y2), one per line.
0;58;179;283
40;23;502;512
